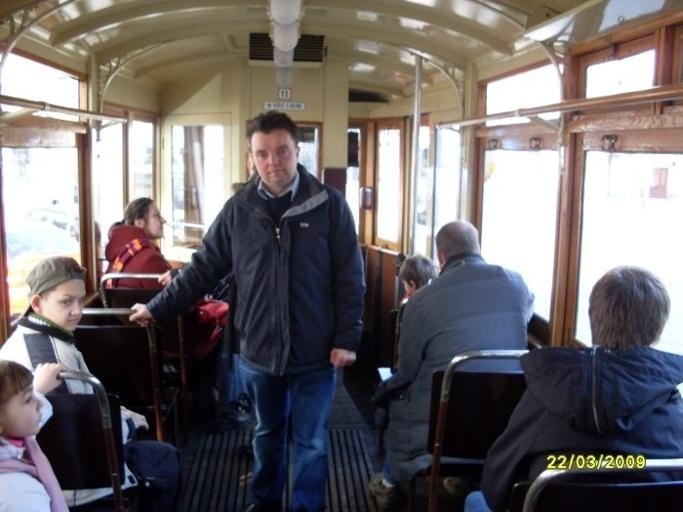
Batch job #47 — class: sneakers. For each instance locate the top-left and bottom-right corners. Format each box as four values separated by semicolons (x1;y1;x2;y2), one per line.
367;473;397;500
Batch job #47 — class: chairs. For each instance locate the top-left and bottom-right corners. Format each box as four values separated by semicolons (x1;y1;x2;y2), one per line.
509;459;683;512
409;350;530;512
73;307;180;446
99;273;202;385
28;369;129;512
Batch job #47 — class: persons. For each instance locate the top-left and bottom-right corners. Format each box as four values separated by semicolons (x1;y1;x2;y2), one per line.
0;359;70;511
368;219;534;510
398;253;437;307
0;254;184;511
103;195;229;423
458;265;682;512
125;109;366;511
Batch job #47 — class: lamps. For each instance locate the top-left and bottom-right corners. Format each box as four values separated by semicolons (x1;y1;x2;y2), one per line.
266;0;304;67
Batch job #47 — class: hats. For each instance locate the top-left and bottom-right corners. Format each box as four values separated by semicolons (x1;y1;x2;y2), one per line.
10;256;86;329
